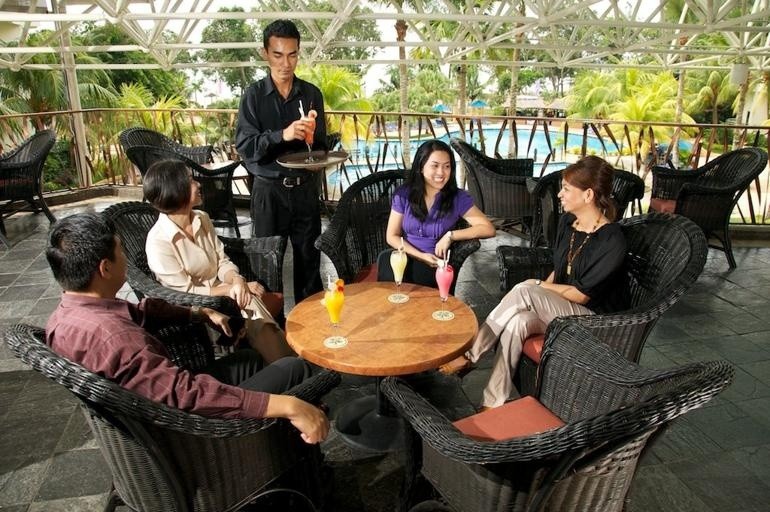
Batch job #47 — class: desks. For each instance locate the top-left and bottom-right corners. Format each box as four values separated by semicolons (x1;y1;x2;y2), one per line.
281;278;480;461
189;155;253;229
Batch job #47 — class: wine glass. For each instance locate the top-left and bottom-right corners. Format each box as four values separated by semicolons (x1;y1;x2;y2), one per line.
323;282;348;349
301;116;318;163
388;251;410;304
432;266;454;321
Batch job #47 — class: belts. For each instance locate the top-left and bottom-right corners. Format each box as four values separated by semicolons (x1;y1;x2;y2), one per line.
254;175;317;188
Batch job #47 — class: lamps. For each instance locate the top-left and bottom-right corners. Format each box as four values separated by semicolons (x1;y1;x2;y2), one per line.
730;62;752;88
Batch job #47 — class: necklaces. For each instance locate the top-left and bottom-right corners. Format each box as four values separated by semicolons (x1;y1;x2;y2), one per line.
566;212;603;275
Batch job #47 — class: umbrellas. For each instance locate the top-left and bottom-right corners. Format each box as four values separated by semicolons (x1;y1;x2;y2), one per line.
433;105;453;120
470;100;487;114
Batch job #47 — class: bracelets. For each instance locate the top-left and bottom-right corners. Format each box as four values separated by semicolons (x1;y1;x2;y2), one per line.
536;279;541;285
446;230;453;242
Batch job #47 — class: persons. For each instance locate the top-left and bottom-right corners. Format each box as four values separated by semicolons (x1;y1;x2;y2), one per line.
40;213;330;446
437;155;633;412
143;158;300;366
234;20;329;307
384;139;496;290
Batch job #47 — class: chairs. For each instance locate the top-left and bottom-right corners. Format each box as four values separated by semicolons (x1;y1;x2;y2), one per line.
376;321;739;511
118;127;216;203
8;308;342;510
125;143;247;236
318;131;344;227
488;208;710;404
93;200;299;370
449;133;537;252
647;145;769;274
531;163;646;247
2;128;57;228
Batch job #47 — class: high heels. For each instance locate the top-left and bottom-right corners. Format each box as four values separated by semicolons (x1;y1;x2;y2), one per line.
438;360;472;377
